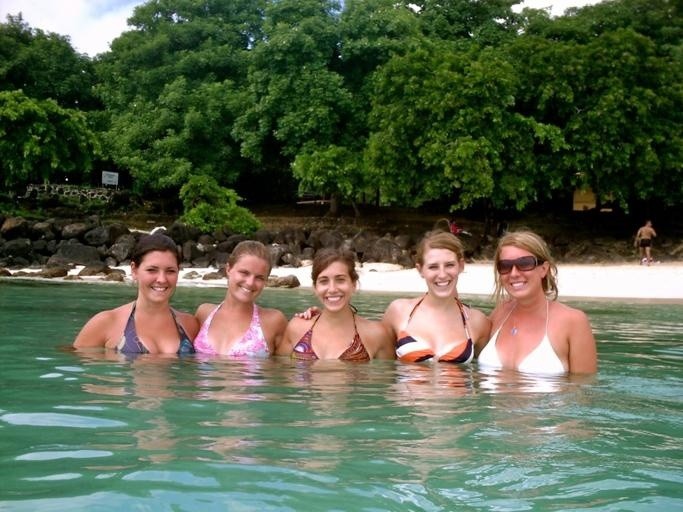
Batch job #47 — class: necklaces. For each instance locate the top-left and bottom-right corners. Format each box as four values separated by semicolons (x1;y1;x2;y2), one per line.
510;303;543;337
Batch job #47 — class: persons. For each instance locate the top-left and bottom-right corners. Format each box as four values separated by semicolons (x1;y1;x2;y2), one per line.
71;231;199;357
449;219;461;237
632;218;659;265
473;229;596;376
272;244;395;362
378;227;491;367
190;241;288;358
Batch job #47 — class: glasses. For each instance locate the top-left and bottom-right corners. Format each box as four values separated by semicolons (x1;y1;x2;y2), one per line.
497;255;544;274
